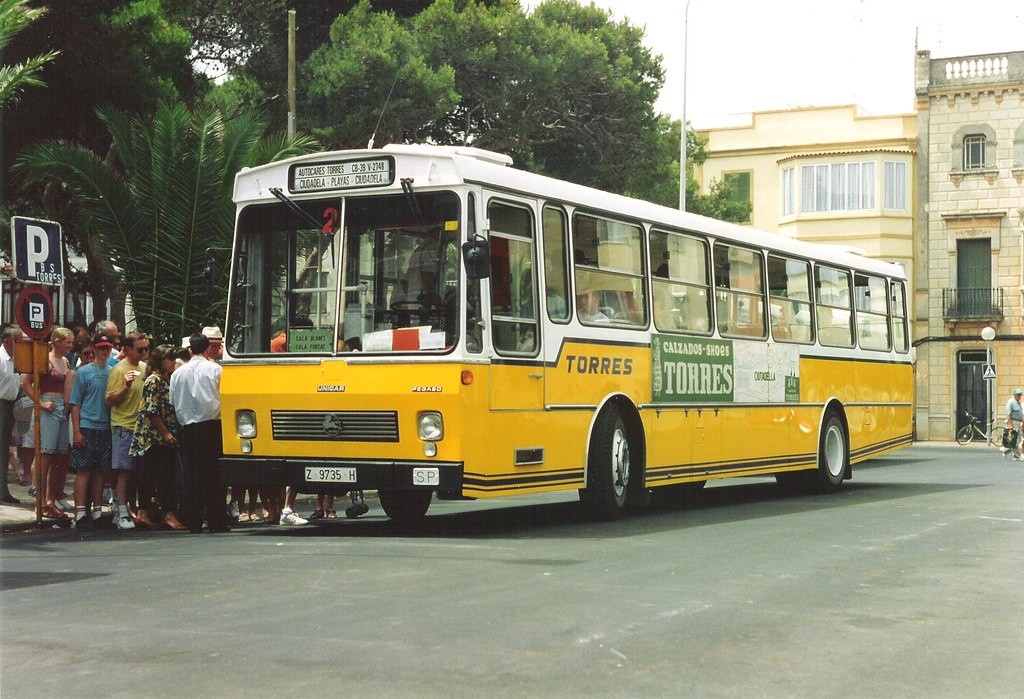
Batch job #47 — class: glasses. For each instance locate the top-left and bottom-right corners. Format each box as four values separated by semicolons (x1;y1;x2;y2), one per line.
137;345;150;354
83;351;94;355
210;345;223;349
113;342;121;347
165;357;176;361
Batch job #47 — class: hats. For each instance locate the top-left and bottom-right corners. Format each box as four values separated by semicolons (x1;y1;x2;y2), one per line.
181;336;191;348
94;333;114;347
1012;389;1022;395
200;327;224;343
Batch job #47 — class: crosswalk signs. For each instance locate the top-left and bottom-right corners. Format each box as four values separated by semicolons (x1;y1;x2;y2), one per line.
982;364;997;380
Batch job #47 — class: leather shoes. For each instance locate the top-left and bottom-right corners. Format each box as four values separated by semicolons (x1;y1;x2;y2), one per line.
134;517;188;529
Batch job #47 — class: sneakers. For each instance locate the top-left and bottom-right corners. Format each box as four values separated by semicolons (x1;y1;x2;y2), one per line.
279;514;308;526
109;513;137;530
227;502;239;517
237;511;261;521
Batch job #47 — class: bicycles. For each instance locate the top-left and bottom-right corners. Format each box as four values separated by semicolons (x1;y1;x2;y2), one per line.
957;411;1006;446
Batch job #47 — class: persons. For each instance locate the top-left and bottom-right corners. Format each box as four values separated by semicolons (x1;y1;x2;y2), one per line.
999;390;1024;460
0;320;338;532
400;218;447;324
577;287;610;323
778;305;848;345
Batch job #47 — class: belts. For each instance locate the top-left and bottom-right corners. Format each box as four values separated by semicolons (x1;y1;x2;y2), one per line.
1010;418;1022;421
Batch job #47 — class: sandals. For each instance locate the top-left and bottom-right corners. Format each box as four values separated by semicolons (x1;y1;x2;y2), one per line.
34;505;68;519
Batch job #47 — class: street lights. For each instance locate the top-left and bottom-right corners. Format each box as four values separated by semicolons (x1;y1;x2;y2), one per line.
982;326;995;446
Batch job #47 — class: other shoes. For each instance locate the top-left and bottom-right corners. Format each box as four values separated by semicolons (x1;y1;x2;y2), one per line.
1013;454;1024;461
190;525;232;534
0;473;139;514
76;516;113;531
1000;447;1008;457
310;509;337;519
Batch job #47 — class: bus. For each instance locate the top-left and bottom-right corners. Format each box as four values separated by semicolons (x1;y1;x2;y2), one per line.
204;143;918;522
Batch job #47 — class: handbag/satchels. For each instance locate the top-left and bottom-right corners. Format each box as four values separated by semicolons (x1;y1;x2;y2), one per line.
1001;428;1017;448
